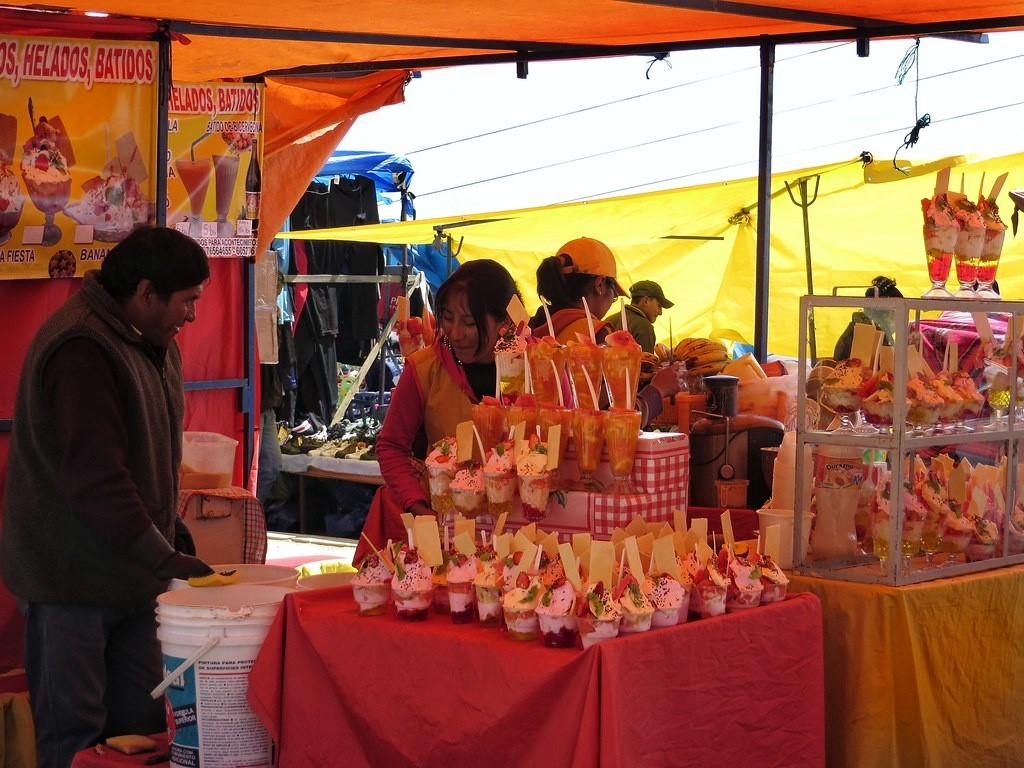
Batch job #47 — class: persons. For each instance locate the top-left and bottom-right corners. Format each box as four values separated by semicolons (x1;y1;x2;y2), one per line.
833;275;904;362
350;259;526;572
0;223;217;768
601;279;674;372
527;236;687;432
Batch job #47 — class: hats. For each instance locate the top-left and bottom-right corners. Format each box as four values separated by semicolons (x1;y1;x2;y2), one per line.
629;279;675;309
555;236;631;300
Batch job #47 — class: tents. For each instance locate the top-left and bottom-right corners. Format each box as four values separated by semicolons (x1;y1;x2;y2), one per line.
310;149;416;298
273;151;1024;360
0;1;1024;701
378;218;463;406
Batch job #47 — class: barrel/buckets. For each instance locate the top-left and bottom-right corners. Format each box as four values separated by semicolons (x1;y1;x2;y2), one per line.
155;584;290;766
702;375;738;420
179;431;240;491
295;575;358;589
169;564;302;592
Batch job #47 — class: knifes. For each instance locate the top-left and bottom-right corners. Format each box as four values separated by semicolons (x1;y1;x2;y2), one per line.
669;317;676;405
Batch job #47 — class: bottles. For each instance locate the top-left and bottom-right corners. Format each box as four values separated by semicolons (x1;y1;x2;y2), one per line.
660;359;689;396
769;430;815;516
244;138;260;231
814;428;865;560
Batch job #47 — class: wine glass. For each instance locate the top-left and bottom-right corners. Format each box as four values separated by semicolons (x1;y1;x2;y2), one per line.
821;355;1024;582
21;172;73;246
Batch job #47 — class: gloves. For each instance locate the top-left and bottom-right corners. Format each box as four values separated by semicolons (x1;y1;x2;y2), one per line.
174;514;197;558
129;522;215;582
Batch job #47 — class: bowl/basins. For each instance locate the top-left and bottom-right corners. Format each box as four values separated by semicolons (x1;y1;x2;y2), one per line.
1;199;25;246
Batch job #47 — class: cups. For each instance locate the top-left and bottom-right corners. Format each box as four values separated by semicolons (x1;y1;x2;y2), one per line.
689;374;705;395
423;336;643;550
924;223;1007;301
719;354;769;384
212;154;240;237
755;509;817;571
352;550;789;650
715;479;752;510
174;157;212;238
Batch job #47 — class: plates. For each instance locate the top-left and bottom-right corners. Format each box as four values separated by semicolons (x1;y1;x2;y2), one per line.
61;198;155;242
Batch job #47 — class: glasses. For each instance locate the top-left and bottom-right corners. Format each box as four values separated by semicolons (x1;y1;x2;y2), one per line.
606;278;619;302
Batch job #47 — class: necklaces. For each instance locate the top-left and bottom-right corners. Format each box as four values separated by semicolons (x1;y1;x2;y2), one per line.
444;334;463;366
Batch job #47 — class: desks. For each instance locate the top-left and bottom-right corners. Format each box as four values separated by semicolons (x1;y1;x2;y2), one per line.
736;538;1023;768
286;586;825;768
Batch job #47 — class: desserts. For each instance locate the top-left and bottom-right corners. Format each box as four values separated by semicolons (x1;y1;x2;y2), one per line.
0;125;151;249
357;196;1024;648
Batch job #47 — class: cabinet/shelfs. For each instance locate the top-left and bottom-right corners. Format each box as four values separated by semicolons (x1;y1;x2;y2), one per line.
794;296;1023;586
